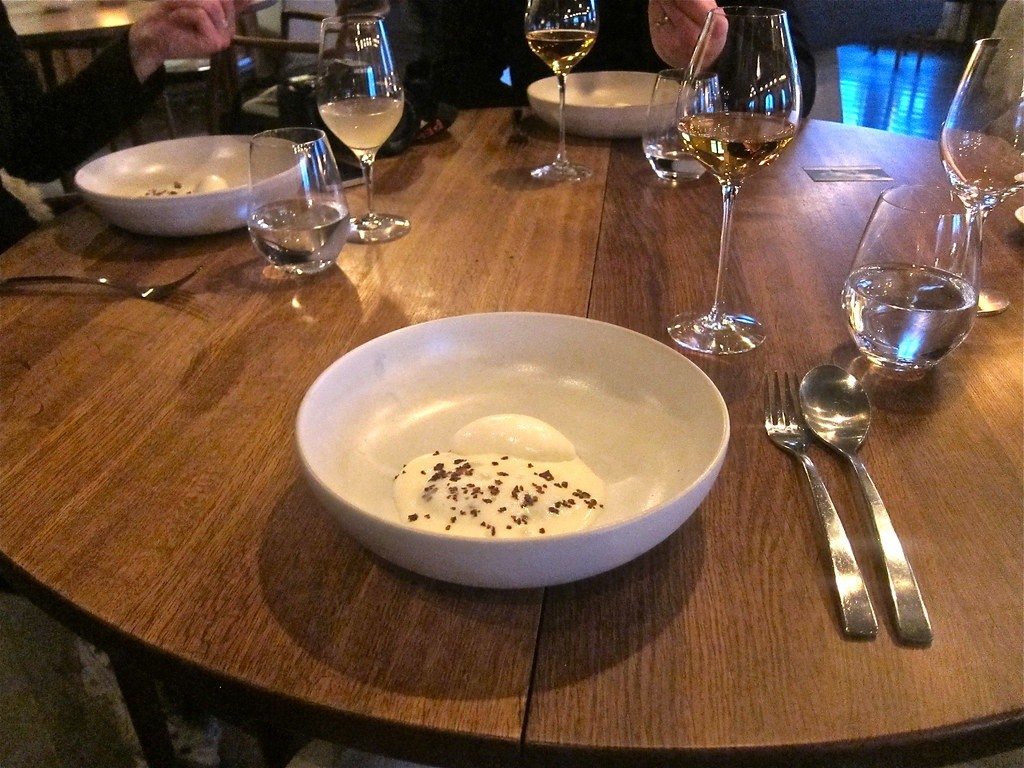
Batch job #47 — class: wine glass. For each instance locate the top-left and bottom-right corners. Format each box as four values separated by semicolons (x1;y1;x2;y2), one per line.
314;15;413;244
524;0;600;184
664;4;803;352
939;35;1024;318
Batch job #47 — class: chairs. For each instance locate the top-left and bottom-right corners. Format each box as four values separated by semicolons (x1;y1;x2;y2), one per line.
206;1;390;133
890;0;977;74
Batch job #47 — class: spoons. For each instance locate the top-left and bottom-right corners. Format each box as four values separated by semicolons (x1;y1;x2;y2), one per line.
797;363;933;645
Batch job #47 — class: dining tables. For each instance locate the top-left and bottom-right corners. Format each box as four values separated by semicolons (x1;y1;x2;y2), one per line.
0;109;1023;768
7;0;262;155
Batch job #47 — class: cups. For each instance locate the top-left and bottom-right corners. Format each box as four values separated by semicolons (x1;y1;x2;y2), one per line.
247;127;351;274
841;185;983;375
643;69;723;182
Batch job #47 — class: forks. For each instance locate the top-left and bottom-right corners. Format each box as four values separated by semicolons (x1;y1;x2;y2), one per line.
764;369;878;637
3;261;206;301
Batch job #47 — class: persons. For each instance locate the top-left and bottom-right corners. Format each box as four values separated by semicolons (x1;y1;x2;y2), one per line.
339;0;817;143
0;0;281;250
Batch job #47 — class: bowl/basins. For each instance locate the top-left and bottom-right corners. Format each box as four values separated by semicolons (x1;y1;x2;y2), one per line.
73;135;307;238
527;71;699;140
293;311;731;590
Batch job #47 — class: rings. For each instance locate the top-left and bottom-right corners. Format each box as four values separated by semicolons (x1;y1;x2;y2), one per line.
655;16;670;26
216;20;229;29
